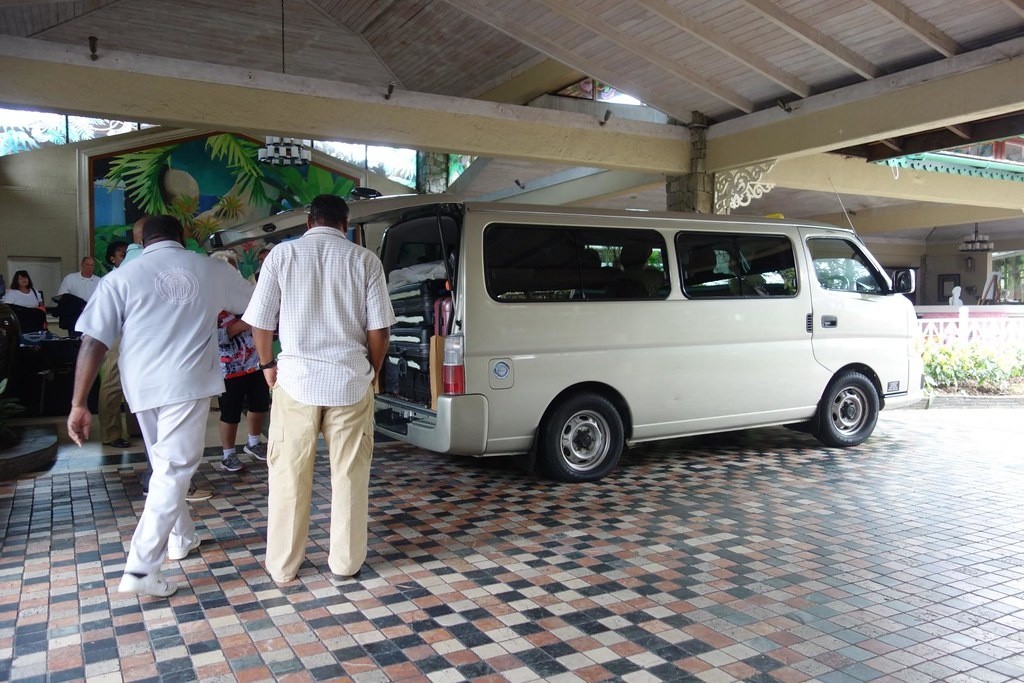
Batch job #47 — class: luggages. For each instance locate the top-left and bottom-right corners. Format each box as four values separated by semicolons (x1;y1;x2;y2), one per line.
384;278;454;409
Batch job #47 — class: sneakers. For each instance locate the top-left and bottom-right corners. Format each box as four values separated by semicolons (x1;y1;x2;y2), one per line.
167;533;201;559
143;488;148;496
244;441;267;460
221;453;246;471
184;480;213;500
118;573;178;596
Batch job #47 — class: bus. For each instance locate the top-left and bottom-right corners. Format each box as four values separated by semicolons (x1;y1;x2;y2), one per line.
209;186;924;482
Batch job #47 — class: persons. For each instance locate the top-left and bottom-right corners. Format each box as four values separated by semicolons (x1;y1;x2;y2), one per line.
254;249;268;281
106;241;127;269
66;214;256;597
97;217;153;450
57;256;102;302
1;270;44;310
211;249;269;472
948;286;963;306
240;192;398;582
